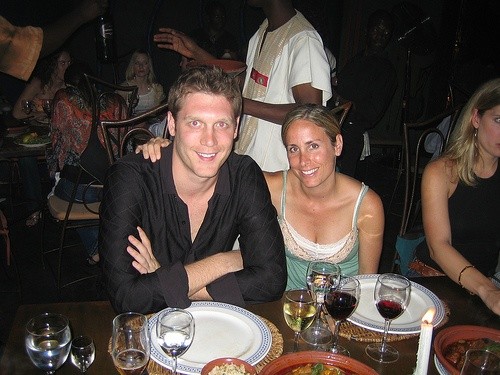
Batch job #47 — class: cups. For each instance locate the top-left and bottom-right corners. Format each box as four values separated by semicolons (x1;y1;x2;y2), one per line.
24;312;72;375
69;335;96;372
460;347;500;375
111;312;150;375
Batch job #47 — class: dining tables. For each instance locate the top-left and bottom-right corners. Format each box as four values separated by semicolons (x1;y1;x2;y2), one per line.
0;290;500;375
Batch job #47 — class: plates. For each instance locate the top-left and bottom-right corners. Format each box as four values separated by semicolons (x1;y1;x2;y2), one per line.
339;274;445;334
13;136;52;147
36;115;51;123
141;302;272;375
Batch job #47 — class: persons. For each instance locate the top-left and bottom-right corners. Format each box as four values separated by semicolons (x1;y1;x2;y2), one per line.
153;0;333;212
0;0;110;81
190;3;239;61
113;47;167;132
458;265;477;289
12;49;73;227
407;77;500;315
135;103;385;297
326;10;398;188
98;64;288;322
45;61;131;267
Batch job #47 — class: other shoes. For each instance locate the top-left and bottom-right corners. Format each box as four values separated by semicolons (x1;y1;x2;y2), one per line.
26;209;42;226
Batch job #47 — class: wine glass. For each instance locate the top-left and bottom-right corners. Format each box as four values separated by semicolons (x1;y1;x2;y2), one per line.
301;259;342;345
22;98;34;124
365;274;411;363
156;308;195;375
282;288;318;354
41;98;54;137
323;274;361;358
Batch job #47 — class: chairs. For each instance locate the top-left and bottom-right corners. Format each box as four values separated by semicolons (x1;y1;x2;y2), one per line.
48;73;458;278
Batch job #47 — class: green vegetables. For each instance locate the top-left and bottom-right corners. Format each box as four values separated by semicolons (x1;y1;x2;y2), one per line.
311;361;324;375
481;338;500;354
14;133;51;144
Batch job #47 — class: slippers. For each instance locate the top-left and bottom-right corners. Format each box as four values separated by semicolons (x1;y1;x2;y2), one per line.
80;257;99;268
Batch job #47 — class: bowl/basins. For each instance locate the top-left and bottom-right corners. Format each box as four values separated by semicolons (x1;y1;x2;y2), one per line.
260;351;380;375
200;357;257;375
435;325;500;375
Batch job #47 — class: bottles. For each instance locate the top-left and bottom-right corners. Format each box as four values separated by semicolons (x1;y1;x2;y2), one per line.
93;9;118;62
